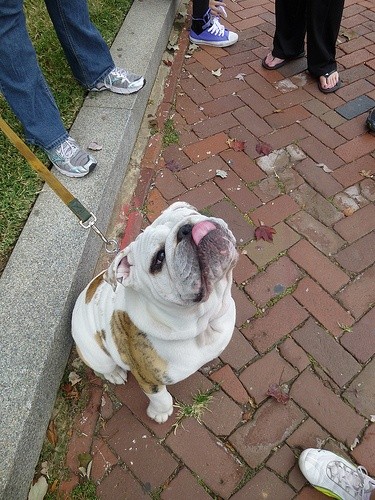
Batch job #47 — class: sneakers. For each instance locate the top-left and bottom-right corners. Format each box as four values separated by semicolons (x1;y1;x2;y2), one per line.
45;136;97;178
298;448;375;500
87;67;146;95
189;8;238;48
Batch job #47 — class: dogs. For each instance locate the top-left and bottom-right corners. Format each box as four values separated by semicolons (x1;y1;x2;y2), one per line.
70;202;240;426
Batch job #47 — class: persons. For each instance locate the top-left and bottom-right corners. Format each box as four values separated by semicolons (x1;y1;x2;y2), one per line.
0;0;145;178
189;0;238;47
262;0;344;93
298;448;375;499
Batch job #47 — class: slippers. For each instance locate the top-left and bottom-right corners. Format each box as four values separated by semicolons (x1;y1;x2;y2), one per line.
318;68;341;93
262;51;305;70
